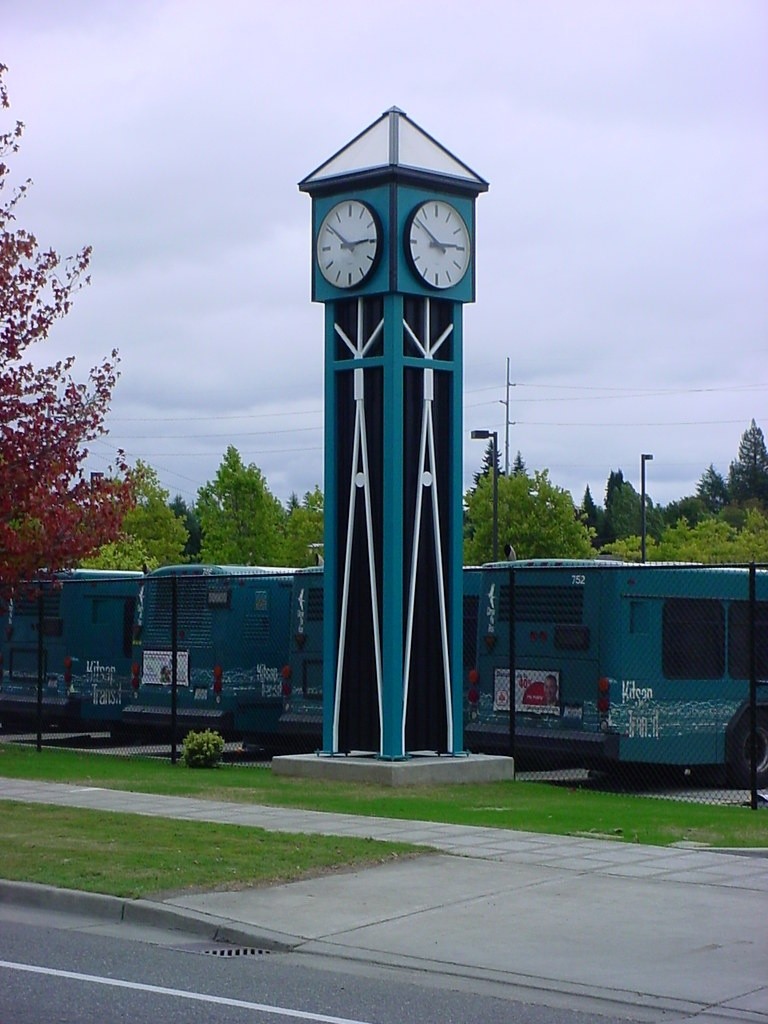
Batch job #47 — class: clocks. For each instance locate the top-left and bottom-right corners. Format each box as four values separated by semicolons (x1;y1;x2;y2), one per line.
403;199;473;291
315;199;383;292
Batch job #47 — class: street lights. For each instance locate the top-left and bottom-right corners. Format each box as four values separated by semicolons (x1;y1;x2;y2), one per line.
468;428;519;757
640;453;656;565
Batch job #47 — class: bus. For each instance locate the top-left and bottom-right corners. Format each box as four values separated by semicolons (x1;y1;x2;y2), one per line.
464;543;768;796
0;567;148;744
120;563;301;743
277;562;485;753
